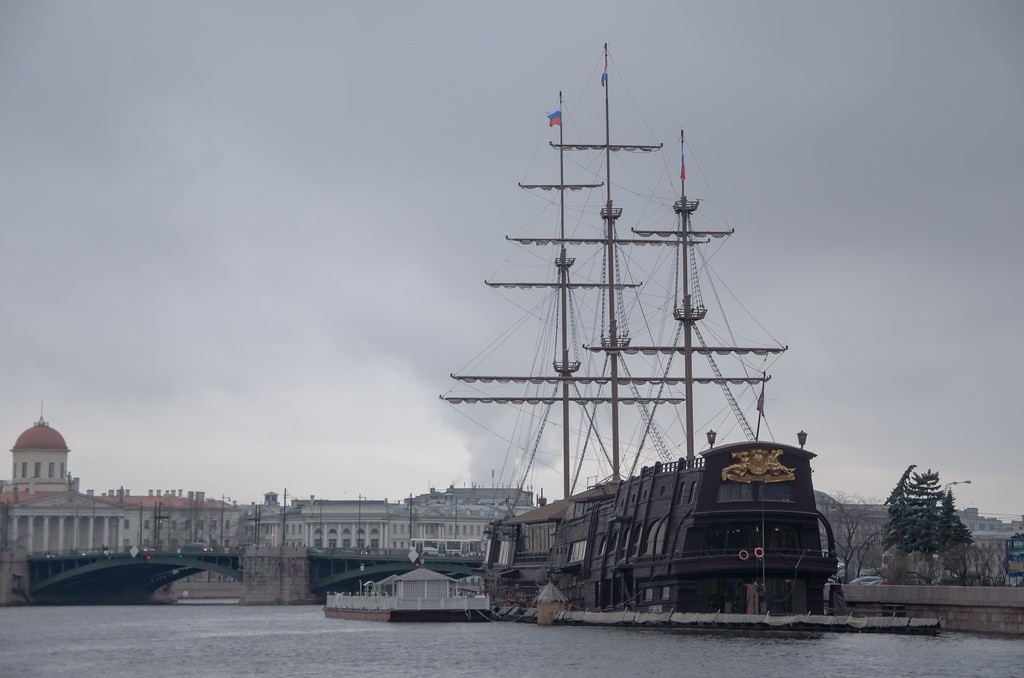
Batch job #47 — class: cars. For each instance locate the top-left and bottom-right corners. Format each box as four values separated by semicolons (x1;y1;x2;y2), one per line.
186;542;214;552
127;544;156;553
849;576;885;586
860;568;881;576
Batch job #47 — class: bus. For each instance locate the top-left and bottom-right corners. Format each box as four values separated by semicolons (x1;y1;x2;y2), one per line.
409;538;482;558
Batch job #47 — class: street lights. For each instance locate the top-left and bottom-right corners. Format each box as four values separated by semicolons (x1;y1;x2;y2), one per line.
219;493;231;547
357;492;367;548
944;479;972;496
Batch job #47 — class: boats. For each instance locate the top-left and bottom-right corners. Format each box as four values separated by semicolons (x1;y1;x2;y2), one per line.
323;565;492;623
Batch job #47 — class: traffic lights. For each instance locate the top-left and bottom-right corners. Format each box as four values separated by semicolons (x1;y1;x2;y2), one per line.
178;554;184;560
145;555;152;560
105;554;113;560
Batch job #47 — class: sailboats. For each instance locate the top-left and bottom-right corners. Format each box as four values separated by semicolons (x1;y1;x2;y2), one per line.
438;41;944;635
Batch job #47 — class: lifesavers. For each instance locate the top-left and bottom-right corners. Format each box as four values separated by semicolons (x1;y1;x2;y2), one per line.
739;550;749;560
754;547;764;557
